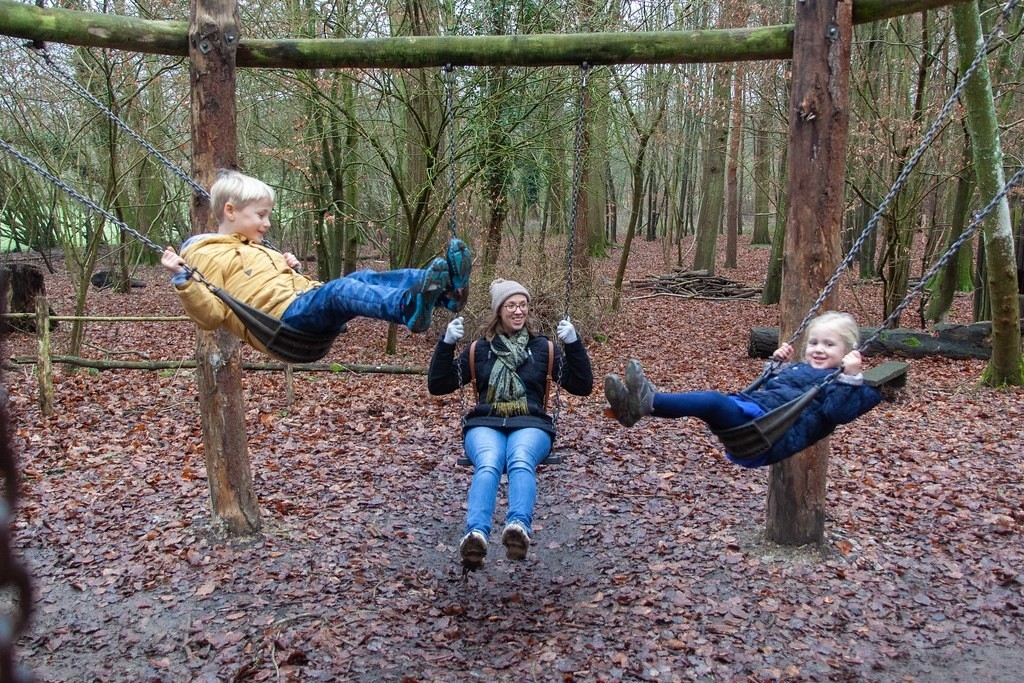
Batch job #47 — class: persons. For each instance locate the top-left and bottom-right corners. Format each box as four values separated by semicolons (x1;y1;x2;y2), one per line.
427;277;594;569
161;168;473;364
604;310;883;468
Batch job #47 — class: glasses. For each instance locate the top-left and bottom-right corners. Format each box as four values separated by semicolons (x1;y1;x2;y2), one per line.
503;301;527;312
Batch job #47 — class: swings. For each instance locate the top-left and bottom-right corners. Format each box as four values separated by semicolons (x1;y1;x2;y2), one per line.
440;63;590;475
716;0;1024;461
0;35;341;363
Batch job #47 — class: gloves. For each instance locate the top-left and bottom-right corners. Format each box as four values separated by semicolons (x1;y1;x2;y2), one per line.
443;317;464;343
557;316;577;343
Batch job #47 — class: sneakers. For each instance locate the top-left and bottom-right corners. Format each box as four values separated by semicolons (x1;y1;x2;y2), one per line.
501;521;531;562
605;376;640;428
625;360;656;416
459;530;488;568
438;239;471;312
398;257;450;331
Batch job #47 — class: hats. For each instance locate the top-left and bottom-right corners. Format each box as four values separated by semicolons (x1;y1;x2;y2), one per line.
488;277;529;314
